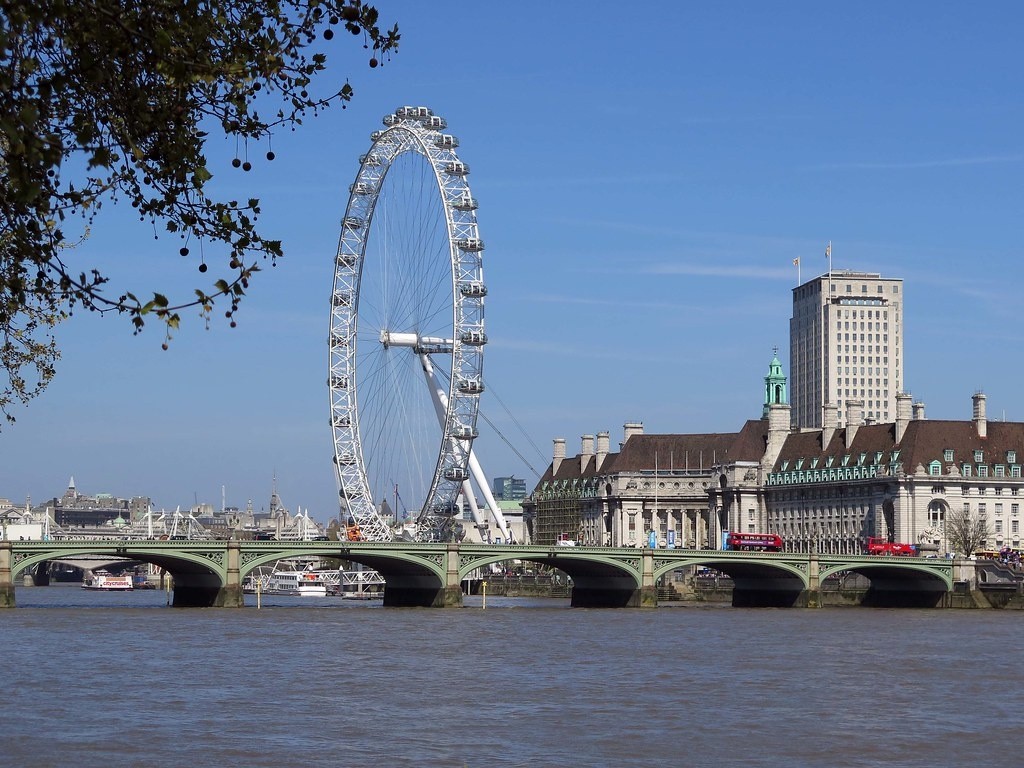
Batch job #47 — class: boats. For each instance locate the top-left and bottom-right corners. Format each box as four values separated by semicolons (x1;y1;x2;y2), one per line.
81;571;156;591
262;569;327;596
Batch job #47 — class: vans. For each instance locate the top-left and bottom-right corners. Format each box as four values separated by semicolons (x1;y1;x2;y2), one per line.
255;536;279;540
170;535;188;540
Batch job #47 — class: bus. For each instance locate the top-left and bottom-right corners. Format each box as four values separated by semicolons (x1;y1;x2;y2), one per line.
865;537;916;556
727;530;785;552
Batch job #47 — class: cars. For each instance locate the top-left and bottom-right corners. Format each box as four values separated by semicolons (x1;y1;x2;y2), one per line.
312;536;330;541
